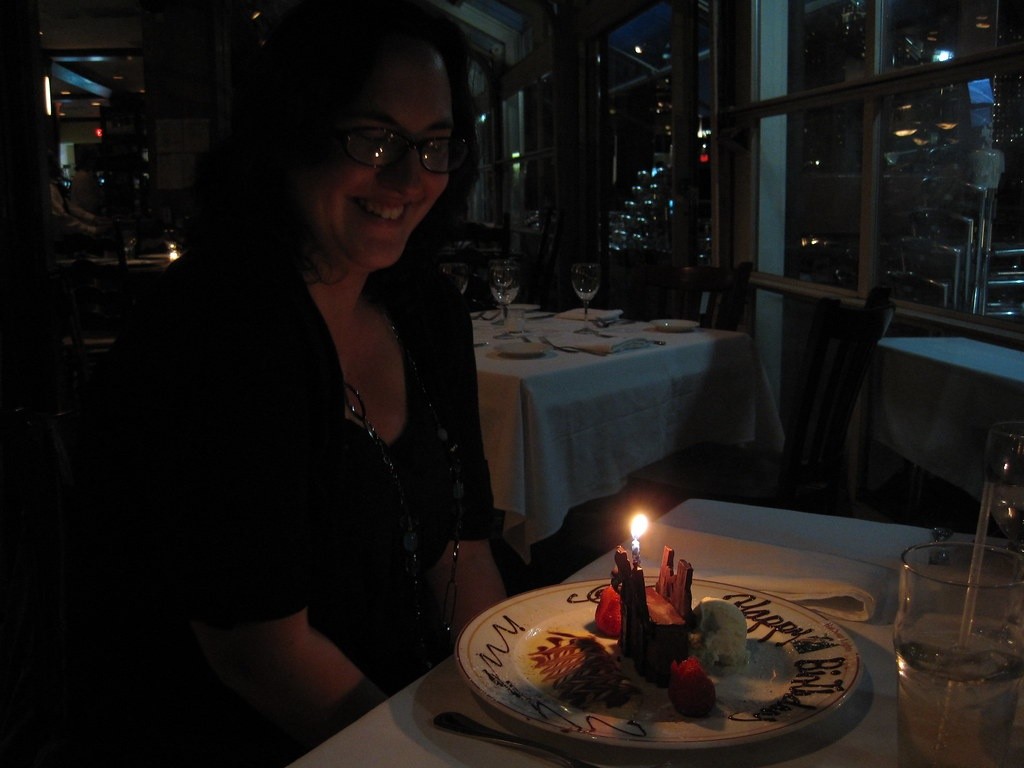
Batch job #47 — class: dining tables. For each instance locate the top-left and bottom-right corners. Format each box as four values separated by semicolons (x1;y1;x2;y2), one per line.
285;498;1024;768
469;311;759;580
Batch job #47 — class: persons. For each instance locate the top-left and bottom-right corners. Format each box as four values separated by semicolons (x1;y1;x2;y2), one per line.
59;0;508;743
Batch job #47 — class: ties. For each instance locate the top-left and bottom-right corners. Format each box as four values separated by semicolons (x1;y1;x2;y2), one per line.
57;183;68;208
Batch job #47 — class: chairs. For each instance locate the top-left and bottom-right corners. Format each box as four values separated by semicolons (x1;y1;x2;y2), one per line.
456;213;897;518
0;215;228;768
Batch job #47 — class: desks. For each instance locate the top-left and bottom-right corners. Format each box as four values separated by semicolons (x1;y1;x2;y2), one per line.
55;246;182;332
368;251;558;304
875;333;1024;547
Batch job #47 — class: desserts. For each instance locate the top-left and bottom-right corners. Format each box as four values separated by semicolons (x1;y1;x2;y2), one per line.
685;596;749;665
611;542;693;675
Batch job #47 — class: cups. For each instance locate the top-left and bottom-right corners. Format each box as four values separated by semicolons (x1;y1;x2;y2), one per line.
121;230;138;259
893;541;1024;768
440;262;469;294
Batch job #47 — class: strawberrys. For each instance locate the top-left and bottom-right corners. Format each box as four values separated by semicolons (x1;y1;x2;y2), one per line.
667;657;716;719
594;585;622;637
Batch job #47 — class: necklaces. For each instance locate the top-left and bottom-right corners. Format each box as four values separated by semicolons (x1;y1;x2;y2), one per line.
342;299;465;666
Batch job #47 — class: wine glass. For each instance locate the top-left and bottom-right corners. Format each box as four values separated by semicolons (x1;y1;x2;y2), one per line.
978;420;1024;642
571;262;602;334
488;259;523;339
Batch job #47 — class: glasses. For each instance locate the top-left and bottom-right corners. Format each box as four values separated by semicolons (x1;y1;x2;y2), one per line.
318;118;468;173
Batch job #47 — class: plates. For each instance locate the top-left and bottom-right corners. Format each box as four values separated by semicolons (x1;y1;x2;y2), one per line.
108;259;162;265
554;305;624;320
651;318;700;332
496;304;541;312
455;576;862;750
484;340;552;356
138;253;182;259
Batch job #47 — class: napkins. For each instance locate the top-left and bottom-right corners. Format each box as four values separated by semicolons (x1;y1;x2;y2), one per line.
556;308;624;325
546;333;647;355
698;575;879;623
